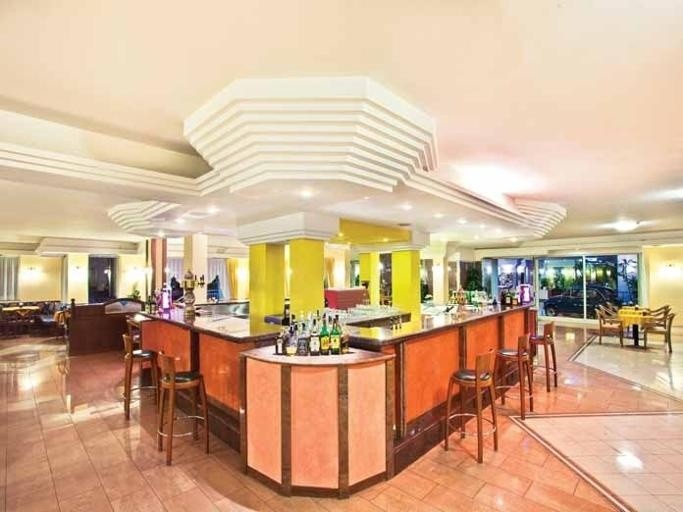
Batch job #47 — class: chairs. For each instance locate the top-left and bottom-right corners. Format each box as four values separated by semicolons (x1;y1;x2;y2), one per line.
496;332;535;420
442;348;498;465
528;319;558;393
121;334;159;420
154;351;209;465
591;299;678;355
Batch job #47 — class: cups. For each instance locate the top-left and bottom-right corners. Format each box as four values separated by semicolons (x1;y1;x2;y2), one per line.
622;301;646;316
158;304;163;314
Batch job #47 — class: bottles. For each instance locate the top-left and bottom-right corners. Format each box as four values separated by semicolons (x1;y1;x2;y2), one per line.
275;296;349;356
504;292;519;306
145;293;157;314
446;289;483;316
389;315;401;330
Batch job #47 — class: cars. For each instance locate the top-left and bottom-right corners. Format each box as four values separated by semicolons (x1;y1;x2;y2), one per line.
541;282;619;318
